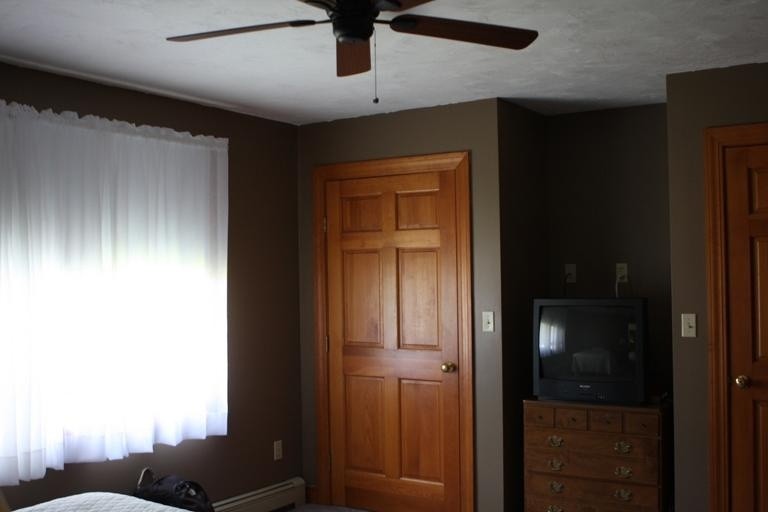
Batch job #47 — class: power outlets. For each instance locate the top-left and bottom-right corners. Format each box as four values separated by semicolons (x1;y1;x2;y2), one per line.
615;262;629;283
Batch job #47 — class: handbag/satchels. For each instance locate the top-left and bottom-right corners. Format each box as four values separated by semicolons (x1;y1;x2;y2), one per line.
133;473;215;512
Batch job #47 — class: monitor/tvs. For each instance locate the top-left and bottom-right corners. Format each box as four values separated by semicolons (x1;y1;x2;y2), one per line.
532;296;649;406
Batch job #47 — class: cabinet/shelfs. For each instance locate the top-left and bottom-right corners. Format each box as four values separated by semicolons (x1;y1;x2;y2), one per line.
521;399;670;510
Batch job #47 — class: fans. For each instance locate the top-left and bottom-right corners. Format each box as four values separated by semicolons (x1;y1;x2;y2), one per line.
166;1;538;79
